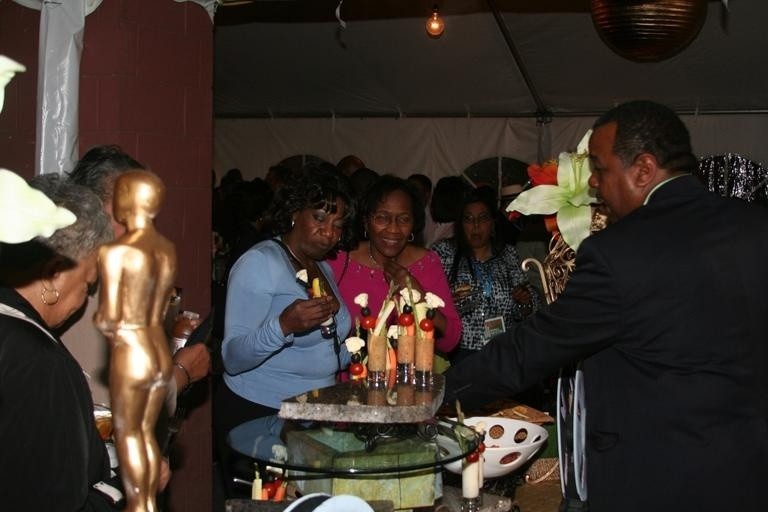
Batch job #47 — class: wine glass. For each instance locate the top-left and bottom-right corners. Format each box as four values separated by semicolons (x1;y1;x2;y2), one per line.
454;276;472;310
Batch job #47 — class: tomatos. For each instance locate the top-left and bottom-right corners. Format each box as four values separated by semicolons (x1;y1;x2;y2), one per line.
349;362;364;375
398;313;413;326
467;451;479;463
263;480;281;498
419;319;435;332
476;442;486;453
361;316;377;330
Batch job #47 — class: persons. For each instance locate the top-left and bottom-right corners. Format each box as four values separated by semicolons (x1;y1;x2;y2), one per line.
429;192;542;360
212;150;377;487
91;168;181;511
440;98;766;509
1;173;173;512
52;141;216;452
317;174;461;351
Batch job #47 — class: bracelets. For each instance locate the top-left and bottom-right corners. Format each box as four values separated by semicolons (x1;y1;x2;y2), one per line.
170;360;193;390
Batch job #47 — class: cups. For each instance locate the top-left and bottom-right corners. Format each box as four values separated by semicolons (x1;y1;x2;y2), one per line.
461;434;485;512
310;282;338;339
365;331;434;391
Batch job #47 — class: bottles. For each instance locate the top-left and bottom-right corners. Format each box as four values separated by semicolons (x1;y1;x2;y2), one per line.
511;270;531;324
167;310;199;359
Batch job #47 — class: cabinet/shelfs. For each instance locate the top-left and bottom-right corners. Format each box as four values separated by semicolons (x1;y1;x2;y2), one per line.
224;372;512;512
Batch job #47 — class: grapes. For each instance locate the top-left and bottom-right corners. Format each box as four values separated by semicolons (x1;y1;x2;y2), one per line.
351;353;361;363
361;307;370;316
403;305;412;312
426;310;435;320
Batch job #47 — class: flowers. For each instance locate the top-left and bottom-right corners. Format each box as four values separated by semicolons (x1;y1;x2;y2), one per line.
503;129;604;255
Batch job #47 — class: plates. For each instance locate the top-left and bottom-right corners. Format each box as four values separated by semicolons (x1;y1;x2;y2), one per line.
429;415;549;480
